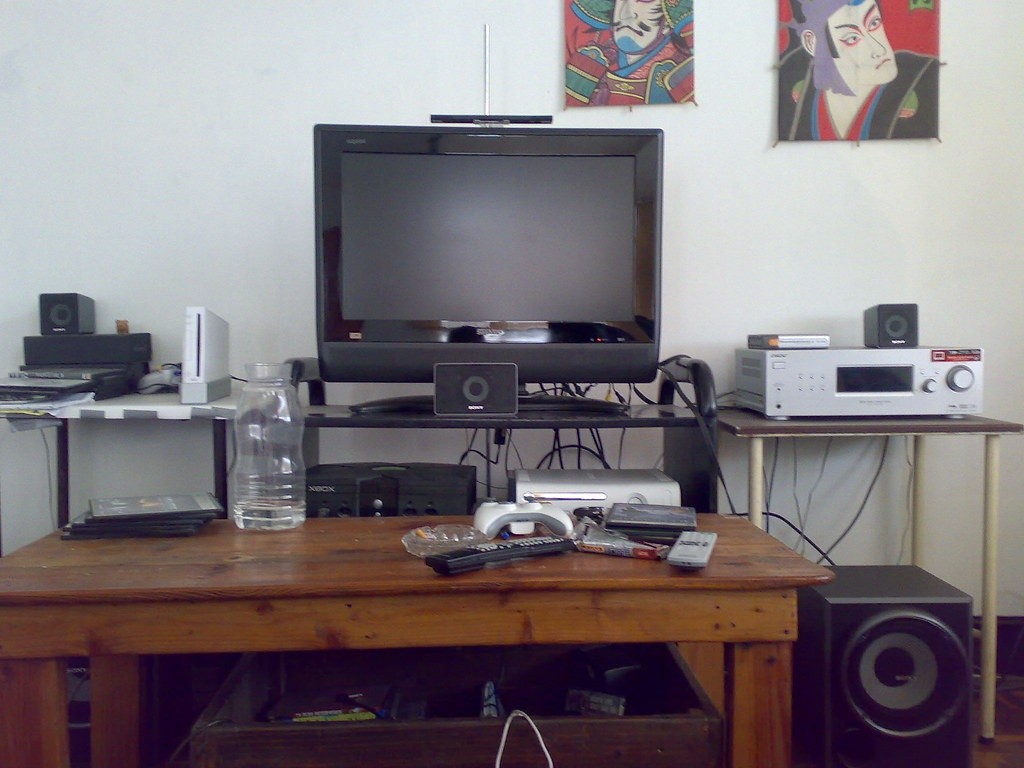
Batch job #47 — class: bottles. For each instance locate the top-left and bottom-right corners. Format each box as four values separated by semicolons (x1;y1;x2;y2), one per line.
232;362;306;531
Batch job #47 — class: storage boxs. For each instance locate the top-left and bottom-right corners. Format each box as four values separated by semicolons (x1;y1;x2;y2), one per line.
180;307;231;402
19;333;151;399
187;641;723;766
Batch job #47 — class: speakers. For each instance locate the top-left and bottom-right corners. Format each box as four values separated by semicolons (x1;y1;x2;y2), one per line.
862;303;919;348
39;293;96;335
791;563;975;768
430;362;518;419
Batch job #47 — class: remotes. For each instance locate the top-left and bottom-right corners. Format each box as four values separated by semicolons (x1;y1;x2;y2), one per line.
424;536;576;571
667;530;718;569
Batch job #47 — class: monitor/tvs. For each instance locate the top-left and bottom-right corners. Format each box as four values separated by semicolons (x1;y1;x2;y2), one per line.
312;122;665;410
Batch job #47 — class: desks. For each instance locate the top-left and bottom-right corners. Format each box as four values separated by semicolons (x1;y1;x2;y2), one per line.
301;402;708;520
0;514;835;767
711;409;1023;744
1;378;241;529
